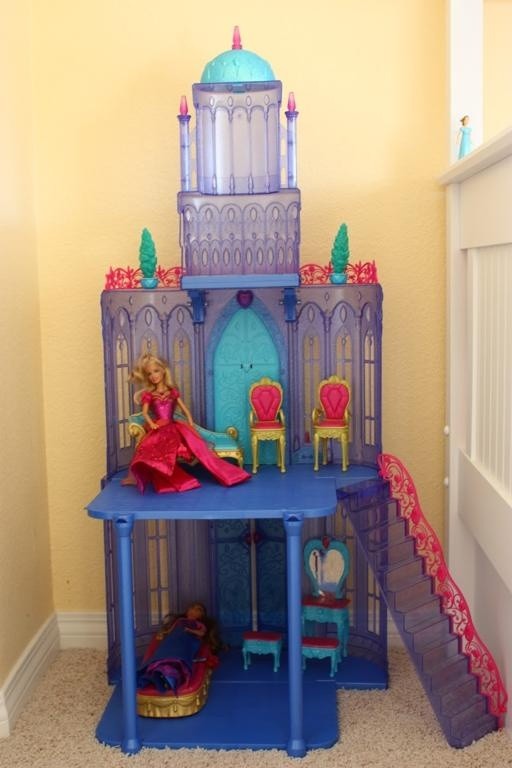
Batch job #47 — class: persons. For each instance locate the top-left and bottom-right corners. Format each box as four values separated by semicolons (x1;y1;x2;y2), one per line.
83;25;507;760
